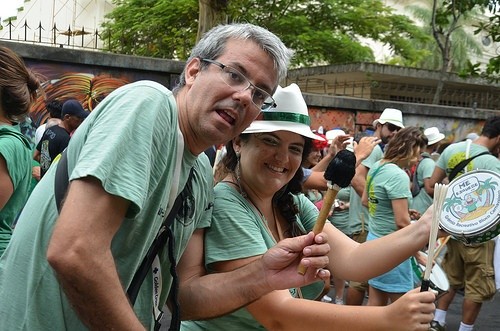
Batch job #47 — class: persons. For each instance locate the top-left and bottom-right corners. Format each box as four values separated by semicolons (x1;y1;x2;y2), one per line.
0;24;331;331
1;47;500;331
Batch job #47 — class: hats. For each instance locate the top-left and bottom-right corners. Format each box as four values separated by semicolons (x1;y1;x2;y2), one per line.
239;82;325;141
326;130;354;144
61;100;90;117
373;108;405;129
424;127;445;145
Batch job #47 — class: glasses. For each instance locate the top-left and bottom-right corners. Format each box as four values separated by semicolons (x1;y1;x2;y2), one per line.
200;59;277;112
385;123;401;132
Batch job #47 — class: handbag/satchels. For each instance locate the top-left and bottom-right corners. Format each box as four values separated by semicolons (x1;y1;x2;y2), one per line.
412;172;420;198
314;199;323;211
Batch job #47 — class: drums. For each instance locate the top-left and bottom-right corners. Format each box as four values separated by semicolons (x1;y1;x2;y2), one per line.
409;251;451;299
434;169;499;245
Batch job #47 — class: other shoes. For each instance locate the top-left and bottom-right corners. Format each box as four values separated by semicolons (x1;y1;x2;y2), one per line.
429;320;448;331
321;295;332;302
335;296;343;304
